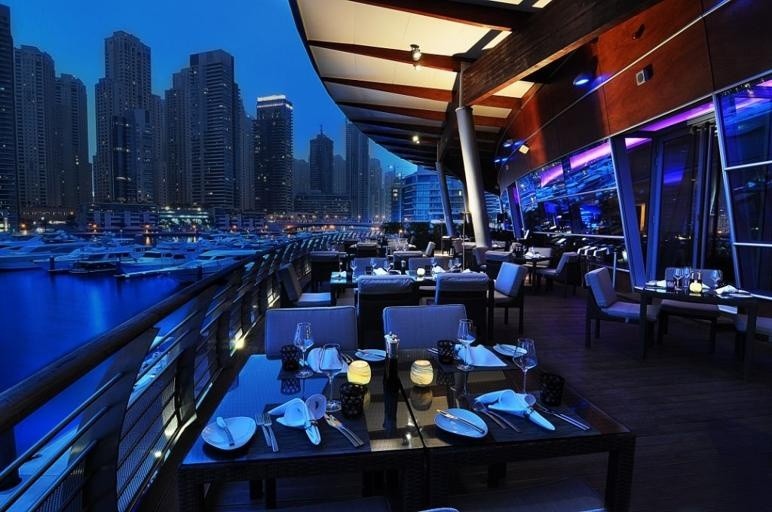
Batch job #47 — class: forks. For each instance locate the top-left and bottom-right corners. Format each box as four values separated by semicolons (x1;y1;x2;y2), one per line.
254;412;272;447
262;413;280;453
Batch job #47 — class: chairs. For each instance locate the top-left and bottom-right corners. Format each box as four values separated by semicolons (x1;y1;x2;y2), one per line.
583;267;770;383
470;242;584;298
279;233;526;347
180;303;635;512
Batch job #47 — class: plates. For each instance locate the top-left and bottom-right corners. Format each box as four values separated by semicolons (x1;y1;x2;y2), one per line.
434;408;489;439
355;349;386;362
492;344;528;358
201;416;257;451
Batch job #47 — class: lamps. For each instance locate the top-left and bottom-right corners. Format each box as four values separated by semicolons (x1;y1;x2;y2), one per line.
572;51;598;86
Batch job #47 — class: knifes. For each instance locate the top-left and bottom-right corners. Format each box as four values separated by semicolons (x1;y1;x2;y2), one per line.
324;413;365;448
534;401;590;431
357;348;386;358
216;416;236;446
495;342;527;355
341;350;355;363
436;409;485;433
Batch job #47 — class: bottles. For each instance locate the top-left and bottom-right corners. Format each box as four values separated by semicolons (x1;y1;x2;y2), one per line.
384;331;399;360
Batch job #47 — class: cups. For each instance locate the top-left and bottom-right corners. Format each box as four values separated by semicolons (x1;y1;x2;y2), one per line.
339;382;367;419
348;360;371;386
410;386;432;411
280;377;302;394
280;345;301;371
472;402;521;432
436;340;455;365
364;390;371;413
537;373;566;407
436;367;455;386
409;360;434;387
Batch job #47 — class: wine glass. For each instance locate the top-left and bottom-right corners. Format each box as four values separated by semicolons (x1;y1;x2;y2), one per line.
457;319;478;372
672;267;721;294
345;257;462;282
293;322;314;378
512;337;539;394
319;343;342;412
